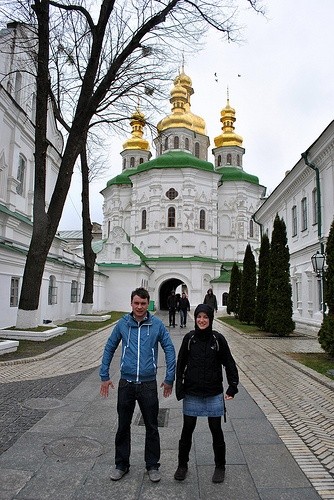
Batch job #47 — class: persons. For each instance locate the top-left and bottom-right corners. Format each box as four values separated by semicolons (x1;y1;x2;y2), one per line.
167;290;179;329
202;288;218;315
177;293;191;328
174;303;240;484
98;288;177;482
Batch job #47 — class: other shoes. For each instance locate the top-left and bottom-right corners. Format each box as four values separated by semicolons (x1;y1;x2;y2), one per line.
184;325;186;327
109;469;125;480
212;467;225;482
147;468;161;482
180;325;183;328
174;464;188;480
